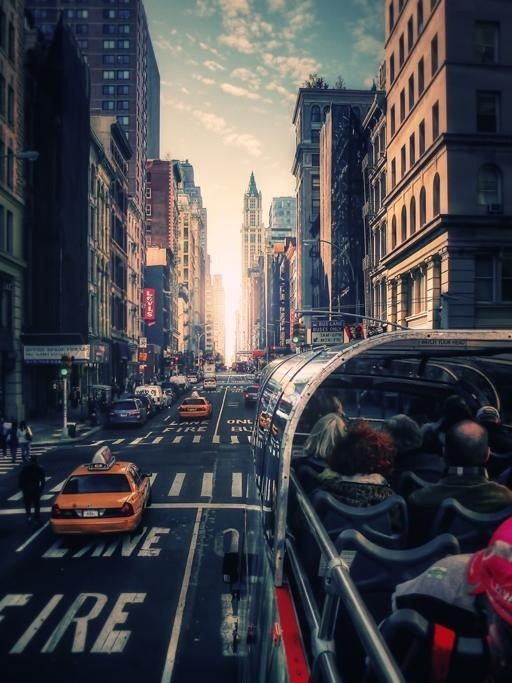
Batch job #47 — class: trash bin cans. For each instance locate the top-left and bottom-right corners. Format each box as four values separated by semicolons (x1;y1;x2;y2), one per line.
67;423;77;438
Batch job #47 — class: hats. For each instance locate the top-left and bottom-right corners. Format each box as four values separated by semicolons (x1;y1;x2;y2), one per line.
476;405;500;417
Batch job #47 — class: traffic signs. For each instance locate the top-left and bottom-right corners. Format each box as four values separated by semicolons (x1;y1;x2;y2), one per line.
310;318;345;332
310;330;344;345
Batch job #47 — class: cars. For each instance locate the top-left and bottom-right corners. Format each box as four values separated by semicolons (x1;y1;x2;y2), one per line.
230;358;251;372
106;371;201;428
203;378;217;390
243;382;261;403
178;389;214;420
50;443;155;539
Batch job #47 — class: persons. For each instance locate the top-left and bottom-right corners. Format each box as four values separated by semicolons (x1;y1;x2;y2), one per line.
1;421;51;524
285;372;511;683
70;371;164;418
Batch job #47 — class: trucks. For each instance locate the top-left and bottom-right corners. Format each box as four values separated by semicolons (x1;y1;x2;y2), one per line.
202;363;218;379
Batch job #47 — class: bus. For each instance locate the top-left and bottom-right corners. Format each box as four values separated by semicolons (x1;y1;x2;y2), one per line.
241;328;512;681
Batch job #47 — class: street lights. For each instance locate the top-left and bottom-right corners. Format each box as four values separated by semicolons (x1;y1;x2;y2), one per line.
302;237;362;328
294;307;415;331
197;329;214;370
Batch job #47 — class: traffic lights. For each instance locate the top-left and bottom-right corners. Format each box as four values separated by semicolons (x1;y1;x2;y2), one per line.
169;357;175;367
174;353;180;362
300;323;305;342
292;323;299;344
58;353;72;381
